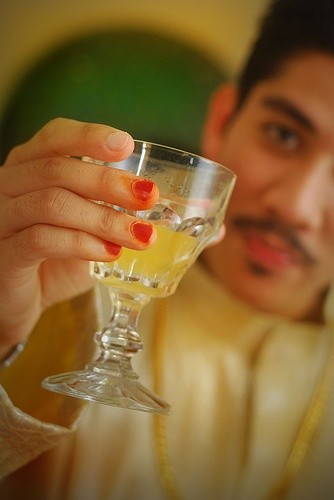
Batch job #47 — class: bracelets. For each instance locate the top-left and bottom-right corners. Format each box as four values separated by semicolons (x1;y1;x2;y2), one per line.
0;336;32;367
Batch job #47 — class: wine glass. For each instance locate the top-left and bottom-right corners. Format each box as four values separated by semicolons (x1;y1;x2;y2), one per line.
39;138;237;414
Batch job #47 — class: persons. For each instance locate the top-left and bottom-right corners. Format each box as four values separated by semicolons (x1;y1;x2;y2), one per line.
1;0;334;500
